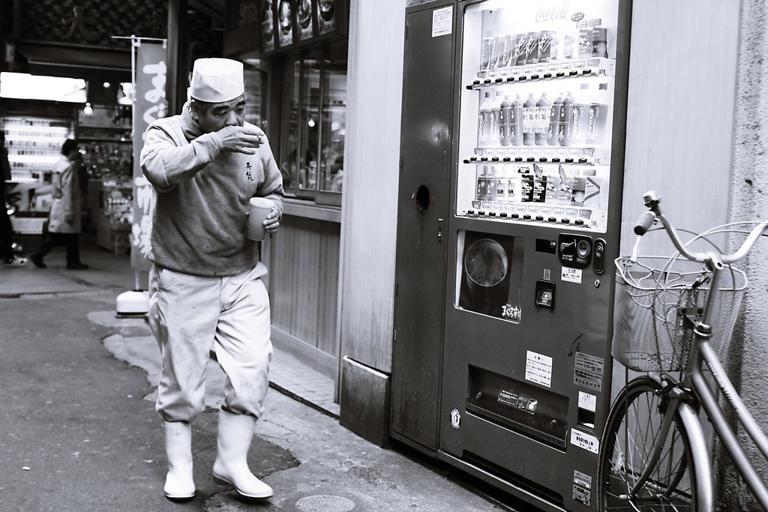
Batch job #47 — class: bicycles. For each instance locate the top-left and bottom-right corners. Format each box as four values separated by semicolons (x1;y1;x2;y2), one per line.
596;191;768;511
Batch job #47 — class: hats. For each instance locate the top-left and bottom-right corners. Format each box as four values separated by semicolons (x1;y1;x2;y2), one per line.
189;57;246;103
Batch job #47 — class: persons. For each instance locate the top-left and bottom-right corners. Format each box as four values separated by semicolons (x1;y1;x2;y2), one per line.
139;58;286;499
71;151;90;233
28;138;90;270
0;130;28;268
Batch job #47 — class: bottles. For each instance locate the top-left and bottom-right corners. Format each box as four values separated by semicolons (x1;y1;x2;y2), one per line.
476;83;612;203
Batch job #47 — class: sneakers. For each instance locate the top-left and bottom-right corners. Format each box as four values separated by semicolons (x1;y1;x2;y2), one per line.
67;261;88;269
5;254;28;267
30;250;47;268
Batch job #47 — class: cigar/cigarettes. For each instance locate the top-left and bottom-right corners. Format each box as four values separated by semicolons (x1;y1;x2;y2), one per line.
255;134;261;140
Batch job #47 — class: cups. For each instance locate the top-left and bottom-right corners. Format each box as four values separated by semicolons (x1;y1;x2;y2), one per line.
246;198;274;242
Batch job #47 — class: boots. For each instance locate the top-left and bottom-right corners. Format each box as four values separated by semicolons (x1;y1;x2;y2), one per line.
161;417;196;499
212;405;275;500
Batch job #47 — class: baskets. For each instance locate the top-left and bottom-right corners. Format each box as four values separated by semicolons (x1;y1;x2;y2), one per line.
611;256;748;373
